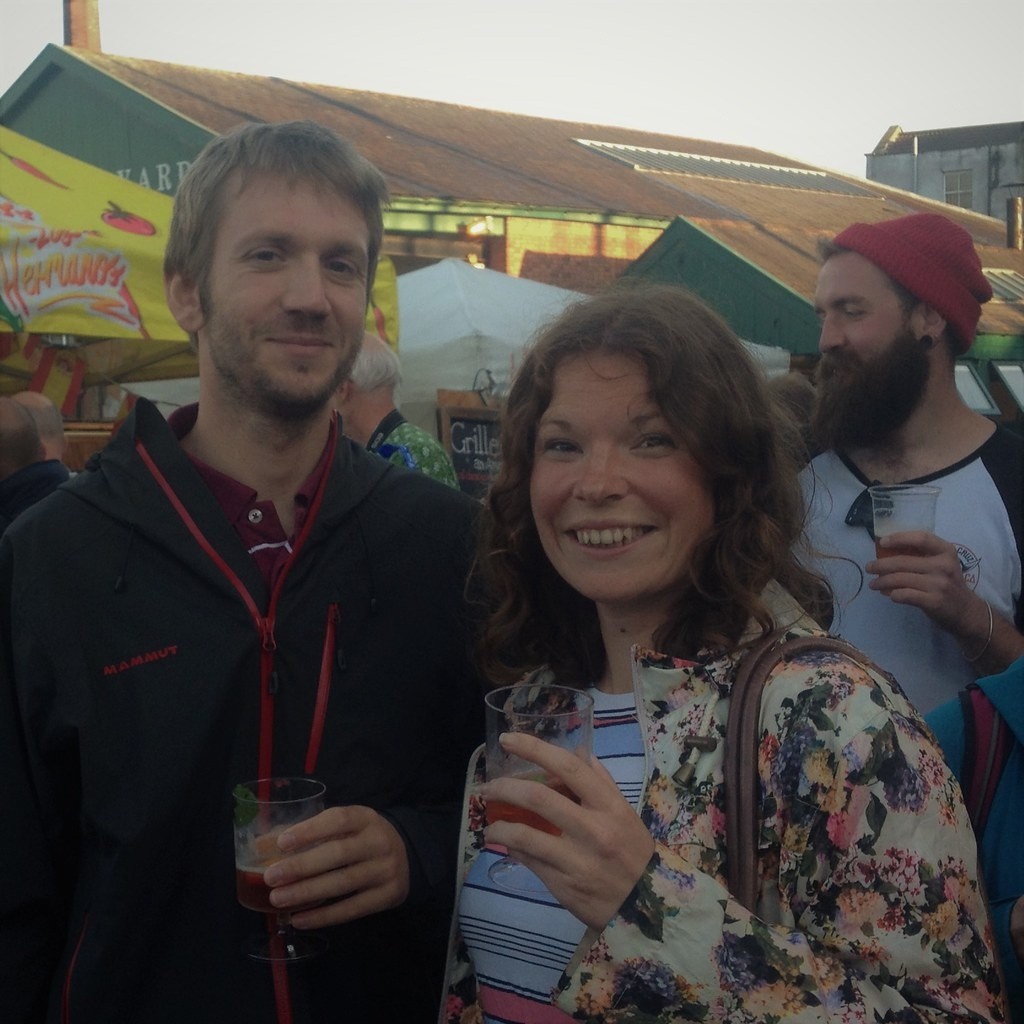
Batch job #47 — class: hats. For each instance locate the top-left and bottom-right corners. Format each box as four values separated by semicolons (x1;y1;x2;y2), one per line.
831;213;994;356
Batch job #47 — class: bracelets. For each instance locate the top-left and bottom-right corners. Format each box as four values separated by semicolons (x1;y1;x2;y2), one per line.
962;599;993;663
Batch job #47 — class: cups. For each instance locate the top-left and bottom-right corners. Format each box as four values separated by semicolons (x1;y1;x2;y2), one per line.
868;484;941;597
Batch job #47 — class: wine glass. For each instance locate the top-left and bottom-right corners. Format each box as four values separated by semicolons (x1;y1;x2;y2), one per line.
231;779;326;963
484;682;594;894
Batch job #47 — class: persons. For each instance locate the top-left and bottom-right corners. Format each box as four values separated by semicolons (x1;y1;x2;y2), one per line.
0;120;501;1024
924;661;1024;1024
438;285;1007;1024
12;392;63;464
337;329;455;489
768;213;1024;716
0;391;70;524
